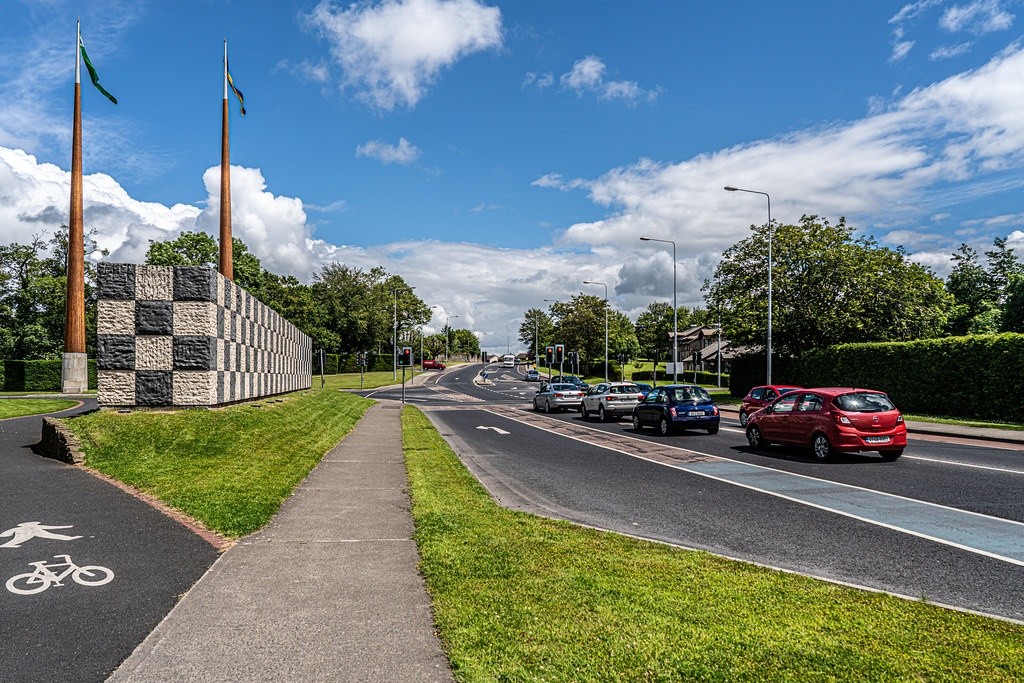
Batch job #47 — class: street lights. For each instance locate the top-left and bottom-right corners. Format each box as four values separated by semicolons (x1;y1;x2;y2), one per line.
394;286;416;381
723;186;771;385
446;316;459;362
544;299;561;303
640;237;677;384
421;306;436;372
583;282;608;383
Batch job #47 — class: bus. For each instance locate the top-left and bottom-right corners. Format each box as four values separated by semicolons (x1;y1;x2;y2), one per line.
504;355;515;367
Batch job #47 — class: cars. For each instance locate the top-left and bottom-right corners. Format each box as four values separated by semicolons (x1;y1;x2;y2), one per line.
739;385;908;464
525;370;659;424
632;384;720;437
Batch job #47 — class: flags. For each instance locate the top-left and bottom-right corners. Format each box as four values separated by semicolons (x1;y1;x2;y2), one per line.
227;57;246;116
80;36;117;104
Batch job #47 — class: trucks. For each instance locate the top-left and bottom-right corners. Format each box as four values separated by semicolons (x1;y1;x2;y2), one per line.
423;360;446;370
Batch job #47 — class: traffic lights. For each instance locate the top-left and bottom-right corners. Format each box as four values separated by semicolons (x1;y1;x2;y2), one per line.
356;351;369;367
397;348;412;366
555;345;564;363
693;352;703;365
568;352;573;364
618;354;621;365
573;352;578;365
546;347;554;364
